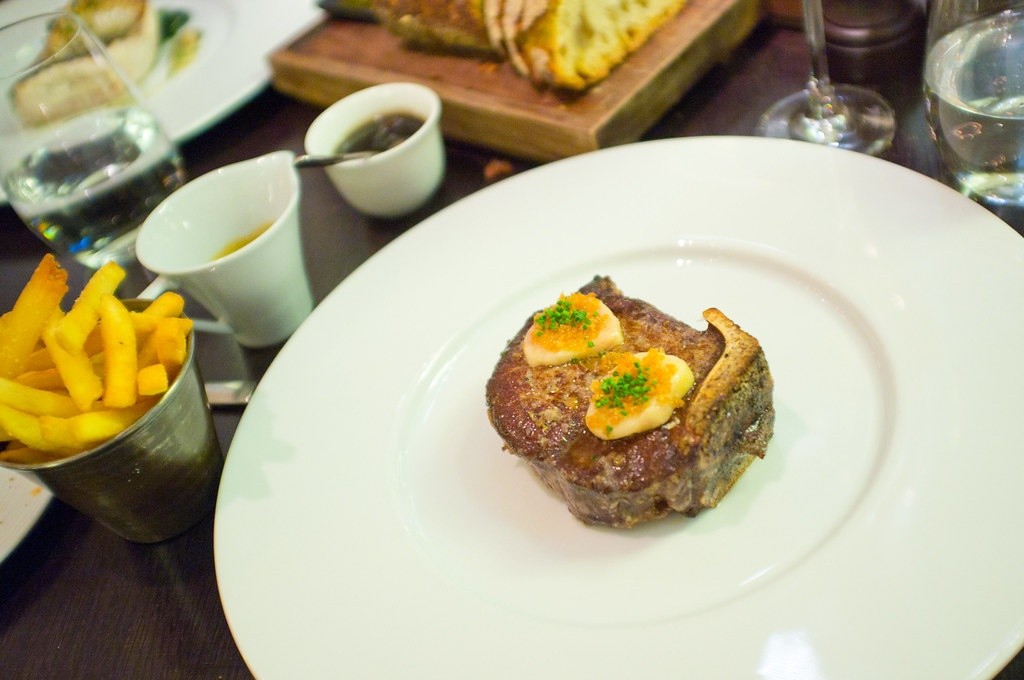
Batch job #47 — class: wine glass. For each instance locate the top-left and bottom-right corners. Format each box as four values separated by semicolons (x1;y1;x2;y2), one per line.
752;0;897;154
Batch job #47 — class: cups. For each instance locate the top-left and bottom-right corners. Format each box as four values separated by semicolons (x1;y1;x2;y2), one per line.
819;0;919;60
921;0;1024;206
134;149;313;348
0;297;225;544
0;11;186;269
304;82;447;219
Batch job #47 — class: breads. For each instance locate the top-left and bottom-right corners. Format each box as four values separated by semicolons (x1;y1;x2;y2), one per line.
364;0;688;94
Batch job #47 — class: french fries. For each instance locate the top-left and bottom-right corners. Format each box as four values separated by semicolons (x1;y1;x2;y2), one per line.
0;252;199;469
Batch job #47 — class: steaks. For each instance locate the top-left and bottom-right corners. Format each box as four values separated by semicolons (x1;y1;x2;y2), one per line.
483;272;777;529
9;3;163;125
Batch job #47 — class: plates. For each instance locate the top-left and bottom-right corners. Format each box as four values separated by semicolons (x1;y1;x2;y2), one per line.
0;2;327;206
1;466;54;561
214;139;1024;680
